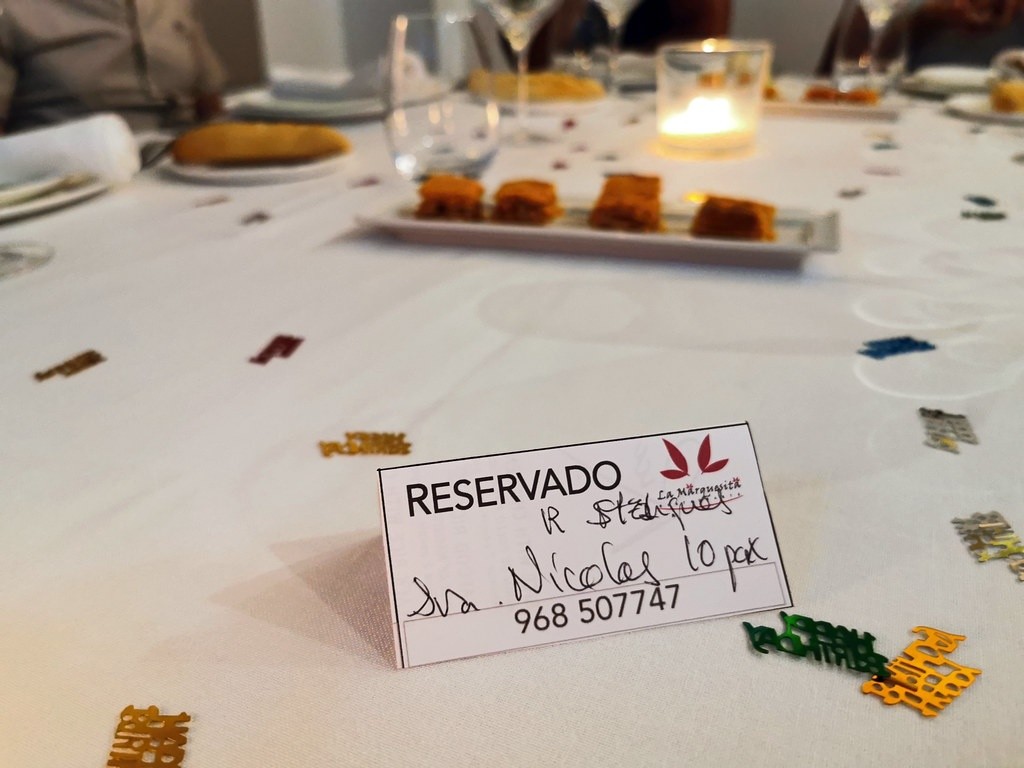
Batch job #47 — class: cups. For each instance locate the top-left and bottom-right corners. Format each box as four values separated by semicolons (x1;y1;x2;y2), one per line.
831;5;908;99
381;12;502;189
654;44;770;163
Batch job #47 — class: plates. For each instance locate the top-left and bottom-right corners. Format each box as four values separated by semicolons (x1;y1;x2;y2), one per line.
158;148;355;187
355;190;813;274
944;93;1024;125
912;62;989;92
763;97;908;122
232;78;450;120
0;174;104;224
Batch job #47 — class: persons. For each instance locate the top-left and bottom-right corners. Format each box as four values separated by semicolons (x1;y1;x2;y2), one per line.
497;0;734;75
814;0;1024;81
0;0;224;132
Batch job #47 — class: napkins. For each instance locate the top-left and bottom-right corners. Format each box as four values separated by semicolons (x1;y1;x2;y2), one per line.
271;53;425;102
0;113;142;190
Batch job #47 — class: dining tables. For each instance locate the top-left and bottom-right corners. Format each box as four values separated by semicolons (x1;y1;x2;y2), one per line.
0;78;1023;768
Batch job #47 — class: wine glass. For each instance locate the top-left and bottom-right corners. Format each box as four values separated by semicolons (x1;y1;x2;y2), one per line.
478;0;563;147
592;0;642;128
857;0;914;78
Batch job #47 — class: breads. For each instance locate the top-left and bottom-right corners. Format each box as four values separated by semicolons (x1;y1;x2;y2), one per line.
415;170;779;243
801;86;878;105
